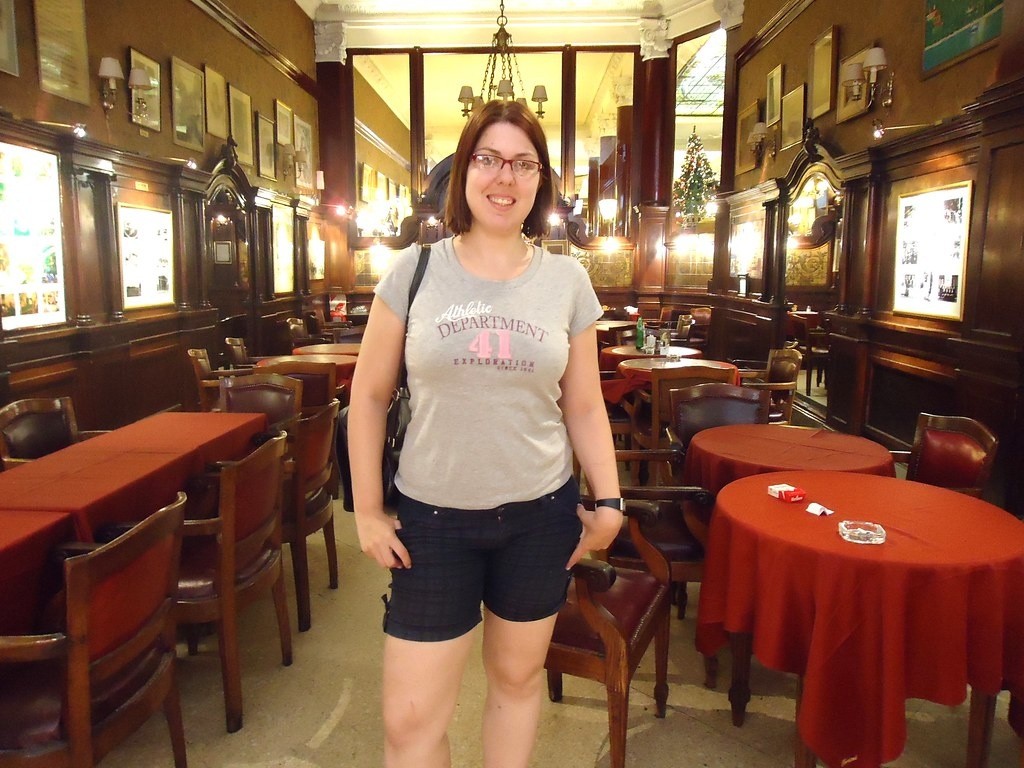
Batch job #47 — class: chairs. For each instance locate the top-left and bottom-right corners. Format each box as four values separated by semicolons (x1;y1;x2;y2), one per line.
284;309;367;352
0;395;114;475
94;338;352;733
0;491;188;768
536;307;998;768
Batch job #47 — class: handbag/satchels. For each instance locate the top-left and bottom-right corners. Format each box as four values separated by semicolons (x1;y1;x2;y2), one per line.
334;387;411;511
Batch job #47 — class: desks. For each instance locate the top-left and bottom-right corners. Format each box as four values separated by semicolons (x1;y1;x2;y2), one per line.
255;343;361;407
592;320;1024;768
0;409;270;641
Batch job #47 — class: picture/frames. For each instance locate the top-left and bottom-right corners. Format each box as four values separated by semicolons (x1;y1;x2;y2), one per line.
917;0;1004;82
779;81;806;151
32;0;92;109
540;239;569;257
832;40;877;125
203;63;230;140
892;179;974;322
736;273;748;298
765;63;783;128
127;45;162;134
806;24;839;121
0;0;19;78
227;80;313;191
171;55;206;153
733;98;760;177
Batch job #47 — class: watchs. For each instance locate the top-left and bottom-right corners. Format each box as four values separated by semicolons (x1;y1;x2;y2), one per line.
595;498;627;512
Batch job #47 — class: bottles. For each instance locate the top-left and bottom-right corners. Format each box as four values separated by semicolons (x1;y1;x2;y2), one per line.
635;316;645;349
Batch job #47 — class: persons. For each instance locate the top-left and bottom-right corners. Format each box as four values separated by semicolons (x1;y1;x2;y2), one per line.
348;103;622;768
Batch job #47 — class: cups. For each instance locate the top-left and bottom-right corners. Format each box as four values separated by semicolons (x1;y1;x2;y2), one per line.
645;331;670;354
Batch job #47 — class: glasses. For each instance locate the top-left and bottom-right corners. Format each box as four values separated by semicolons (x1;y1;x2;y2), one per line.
469;153;543;176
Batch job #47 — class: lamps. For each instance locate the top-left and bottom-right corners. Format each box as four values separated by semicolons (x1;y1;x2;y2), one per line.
747;122;776;163
842;46;895;117
458;0;548;120
282;144;307;181
